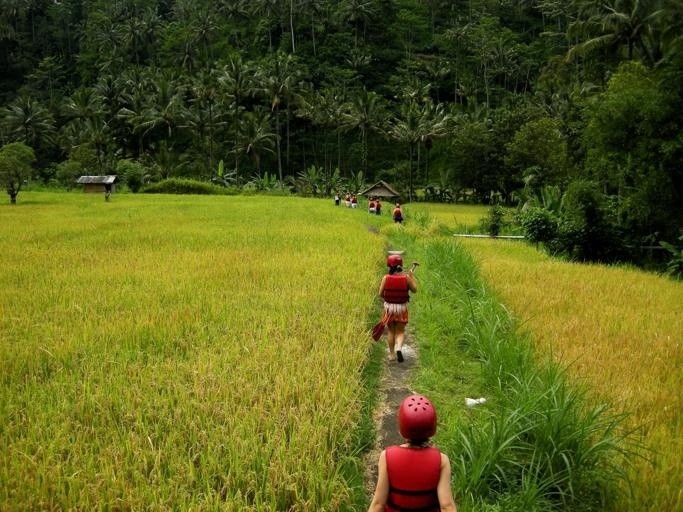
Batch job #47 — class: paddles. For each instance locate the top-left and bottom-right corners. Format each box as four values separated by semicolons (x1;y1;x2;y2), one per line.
371;262;418;341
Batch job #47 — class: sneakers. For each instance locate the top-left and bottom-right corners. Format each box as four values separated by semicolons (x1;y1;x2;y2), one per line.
397;351;403;362
387;354;395;361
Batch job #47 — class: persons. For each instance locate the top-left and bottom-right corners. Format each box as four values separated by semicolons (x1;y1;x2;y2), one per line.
105;191;112;201
367;395;457;512
368;197;376;213
378;255;417;362
393;203;403;223
335;192;358;209
376;200;382;215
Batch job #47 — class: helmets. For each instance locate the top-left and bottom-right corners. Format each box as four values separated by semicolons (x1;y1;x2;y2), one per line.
399;394;437;440
387;254;402;266
